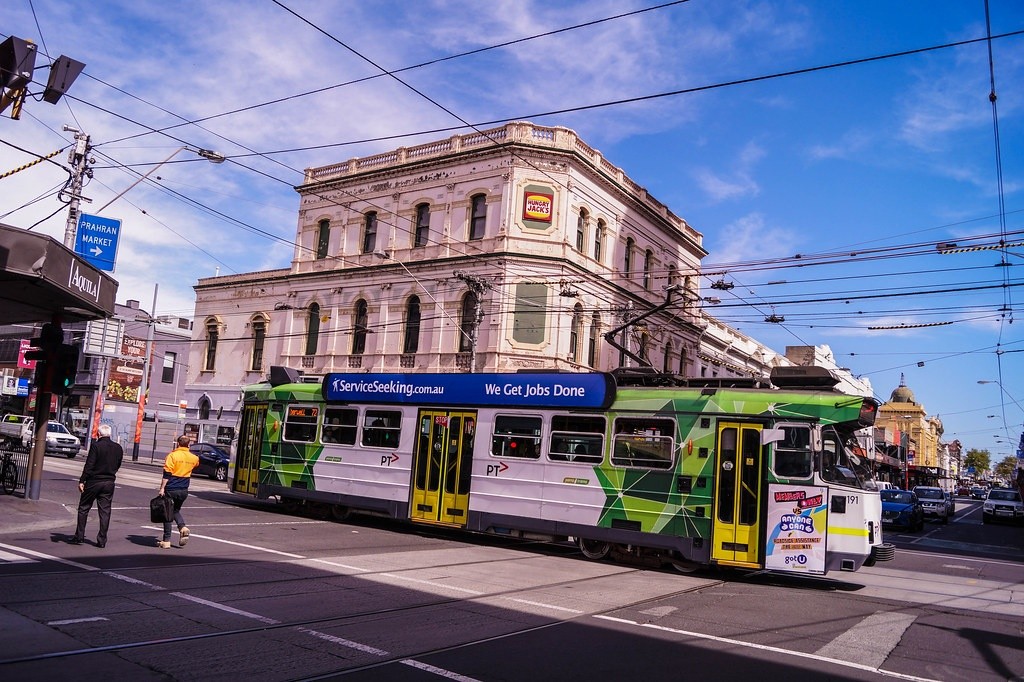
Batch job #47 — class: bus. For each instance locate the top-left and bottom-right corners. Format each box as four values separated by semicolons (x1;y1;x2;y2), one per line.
226;365;895;581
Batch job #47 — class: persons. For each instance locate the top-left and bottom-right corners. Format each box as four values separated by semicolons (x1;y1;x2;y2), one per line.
816;449;846;481
529;440;593;462
322;427;337;443
154;435;198;548
68;425;123;547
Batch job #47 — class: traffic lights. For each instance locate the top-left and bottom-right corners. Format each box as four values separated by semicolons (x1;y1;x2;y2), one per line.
510;437;517;454
51;344;79;396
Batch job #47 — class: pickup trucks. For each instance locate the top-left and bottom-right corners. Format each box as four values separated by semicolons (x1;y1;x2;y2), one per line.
0;414;80;458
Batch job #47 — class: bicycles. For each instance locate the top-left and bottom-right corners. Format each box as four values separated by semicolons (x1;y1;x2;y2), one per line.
0;453;18;495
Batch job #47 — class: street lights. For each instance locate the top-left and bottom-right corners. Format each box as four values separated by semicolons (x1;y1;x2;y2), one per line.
872;415;911;479
373;248;476;373
24;145;225;500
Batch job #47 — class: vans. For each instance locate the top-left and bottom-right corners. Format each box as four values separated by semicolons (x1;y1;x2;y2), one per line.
866;481;892;492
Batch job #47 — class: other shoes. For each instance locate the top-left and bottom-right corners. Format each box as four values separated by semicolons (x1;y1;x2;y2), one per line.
67;536;83;543
97;544;106;547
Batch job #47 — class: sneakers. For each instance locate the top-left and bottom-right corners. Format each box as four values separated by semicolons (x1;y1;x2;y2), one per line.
156;541;171;549
179;526;190;546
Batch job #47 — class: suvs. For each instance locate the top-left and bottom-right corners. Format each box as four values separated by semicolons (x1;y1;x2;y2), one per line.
983;488;1024;525
913;485;948;523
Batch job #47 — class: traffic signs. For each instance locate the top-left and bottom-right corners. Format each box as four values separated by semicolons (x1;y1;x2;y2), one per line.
73;212;122;272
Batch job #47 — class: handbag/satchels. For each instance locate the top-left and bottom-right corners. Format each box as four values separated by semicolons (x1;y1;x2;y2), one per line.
150;493;174;523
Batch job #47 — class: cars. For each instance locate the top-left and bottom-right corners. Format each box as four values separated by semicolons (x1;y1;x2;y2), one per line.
188;443;231;481
879;489;924;530
946;491;955;517
959;486;987;499
